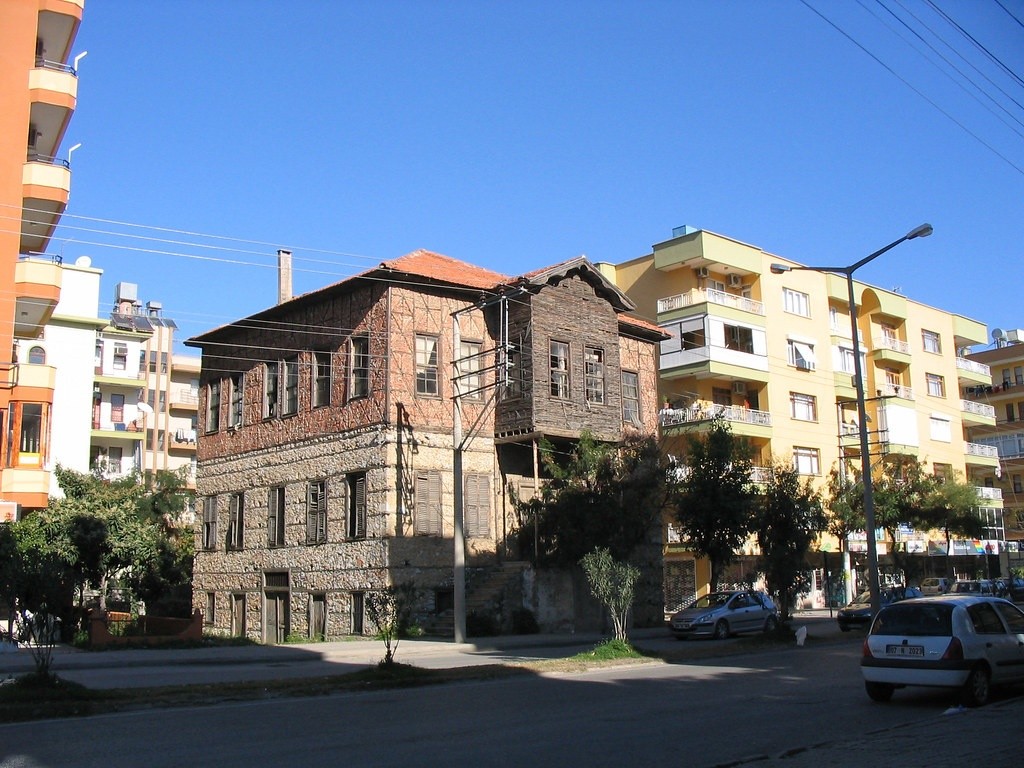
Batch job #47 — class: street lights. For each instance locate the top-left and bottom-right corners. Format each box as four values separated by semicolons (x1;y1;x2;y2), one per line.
769;224;935;623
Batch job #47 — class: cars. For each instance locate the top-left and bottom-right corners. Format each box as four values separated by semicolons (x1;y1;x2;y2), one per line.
921;577;953;597
671;588;779;643
942;578;1013;604
997;576;1024;600
859;596;1024;709
837;586;925;632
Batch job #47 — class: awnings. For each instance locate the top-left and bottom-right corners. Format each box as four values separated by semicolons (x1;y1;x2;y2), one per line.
795;343;817;362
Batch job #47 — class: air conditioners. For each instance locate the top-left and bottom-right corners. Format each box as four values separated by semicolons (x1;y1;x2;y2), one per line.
730;380;747;396
696;267;710;278
726;273;743;290
